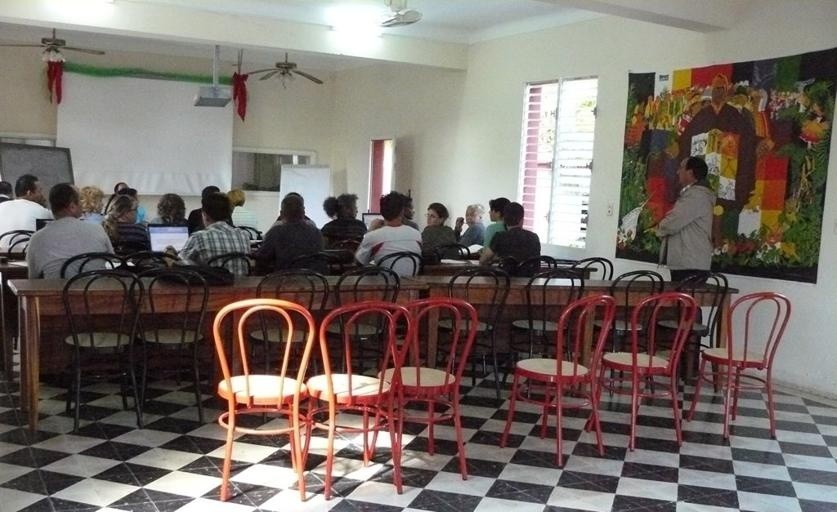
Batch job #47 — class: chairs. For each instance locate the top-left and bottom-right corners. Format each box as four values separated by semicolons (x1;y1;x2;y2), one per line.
686;292;790;440
584;292;697;451
213;299;316;502
369;297;480;481
302;300;415;500
499;292;617;468
0;223;740;430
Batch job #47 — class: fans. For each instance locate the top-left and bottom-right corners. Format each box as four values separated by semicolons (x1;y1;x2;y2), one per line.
0;29;105;61
245;52;324;86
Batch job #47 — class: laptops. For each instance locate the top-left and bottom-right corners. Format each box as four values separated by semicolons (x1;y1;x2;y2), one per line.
147;223;190;255
36;218;89;232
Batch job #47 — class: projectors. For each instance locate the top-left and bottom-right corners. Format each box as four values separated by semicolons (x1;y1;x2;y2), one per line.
192;86;233;108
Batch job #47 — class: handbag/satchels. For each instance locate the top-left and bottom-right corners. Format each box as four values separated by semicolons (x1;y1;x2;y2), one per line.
156;264;234;287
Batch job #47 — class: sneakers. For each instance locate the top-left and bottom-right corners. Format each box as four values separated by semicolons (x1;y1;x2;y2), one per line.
684;378;698;386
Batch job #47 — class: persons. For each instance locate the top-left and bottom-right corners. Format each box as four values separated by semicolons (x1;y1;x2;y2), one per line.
654;155;715;387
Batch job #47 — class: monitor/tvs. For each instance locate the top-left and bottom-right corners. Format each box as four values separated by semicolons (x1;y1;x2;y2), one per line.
362;213;385;231
